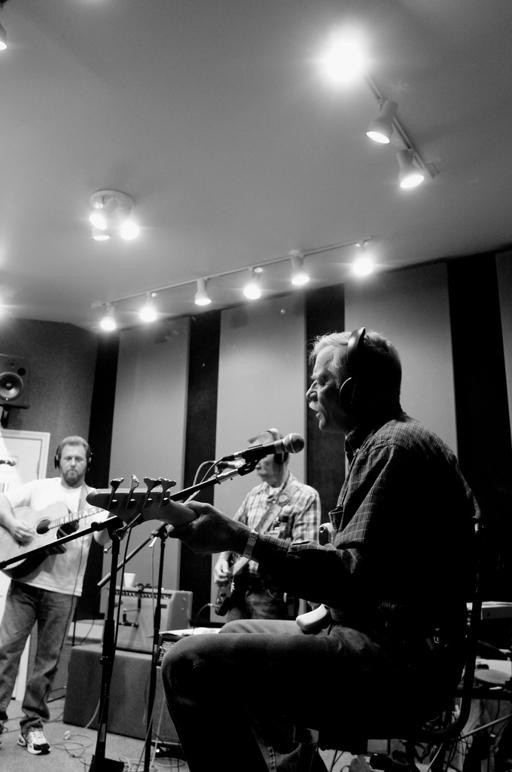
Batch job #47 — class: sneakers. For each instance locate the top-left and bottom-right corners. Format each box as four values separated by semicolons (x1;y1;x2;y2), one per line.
18;725;50;755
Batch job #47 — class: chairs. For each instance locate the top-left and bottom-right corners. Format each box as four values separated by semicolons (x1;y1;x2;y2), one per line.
266;534;494;772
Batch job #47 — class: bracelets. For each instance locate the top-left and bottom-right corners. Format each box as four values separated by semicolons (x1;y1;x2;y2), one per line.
243;528;259;560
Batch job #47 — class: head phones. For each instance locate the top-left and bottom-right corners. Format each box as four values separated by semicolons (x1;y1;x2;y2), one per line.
337;326;381;408
267;427;289;462
54;434;94;474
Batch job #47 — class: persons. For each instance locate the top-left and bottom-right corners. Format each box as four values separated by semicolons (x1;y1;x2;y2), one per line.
213;430;322;623
160;330;485;772
0;436;123;755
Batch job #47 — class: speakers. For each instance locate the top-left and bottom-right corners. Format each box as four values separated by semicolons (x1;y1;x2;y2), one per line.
0;351;35;410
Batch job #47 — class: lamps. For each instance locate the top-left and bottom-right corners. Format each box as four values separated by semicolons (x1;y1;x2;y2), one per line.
365;74;436;189
91;233;376;333
88;188;142;243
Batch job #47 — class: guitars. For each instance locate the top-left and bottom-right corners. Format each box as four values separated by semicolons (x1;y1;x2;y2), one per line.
216;551;252;614
90;474;332;632
0;505;110;577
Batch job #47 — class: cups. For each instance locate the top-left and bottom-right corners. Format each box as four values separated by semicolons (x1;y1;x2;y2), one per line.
122;572;135;589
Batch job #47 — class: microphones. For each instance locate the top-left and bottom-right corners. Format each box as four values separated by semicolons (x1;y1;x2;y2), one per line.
207;458;246;469
221;432;306;461
